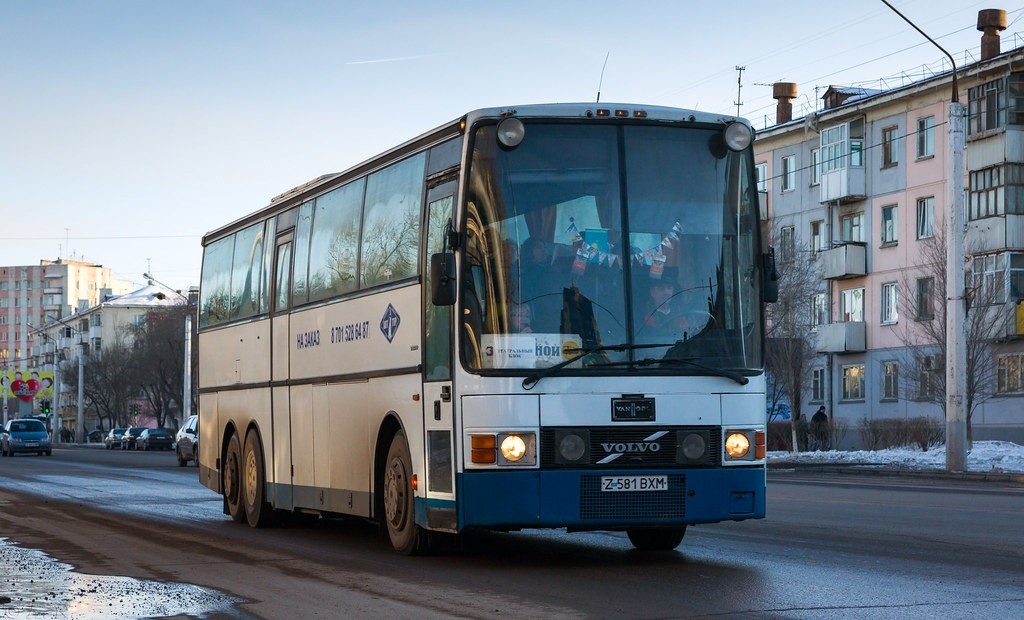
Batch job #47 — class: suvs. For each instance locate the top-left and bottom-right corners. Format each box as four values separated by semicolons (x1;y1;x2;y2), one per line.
175;415;199;467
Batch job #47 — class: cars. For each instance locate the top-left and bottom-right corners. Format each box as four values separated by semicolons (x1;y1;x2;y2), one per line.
104;428;128;450
135;428;174;451
0;419;51;457
120;428;149;451
85;430;109;443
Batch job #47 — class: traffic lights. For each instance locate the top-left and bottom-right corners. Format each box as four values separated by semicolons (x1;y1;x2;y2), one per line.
40;400;45;414
134;404;138;416
139;404;142;414
129;404;133;416
45;401;50;414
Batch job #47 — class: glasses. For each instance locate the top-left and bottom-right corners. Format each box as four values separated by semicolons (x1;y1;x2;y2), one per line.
510;312;530;319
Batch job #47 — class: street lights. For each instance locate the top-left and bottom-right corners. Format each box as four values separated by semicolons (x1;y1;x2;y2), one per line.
27;323;61;444
143;273;193;428
46;313;85;445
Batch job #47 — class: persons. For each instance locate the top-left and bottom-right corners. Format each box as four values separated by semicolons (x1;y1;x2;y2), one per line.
502;237;523;282
60;425;67;441
810;404;830;451
799;413;812;450
510;234;552;302
507;300;534;334
71;428;76;441
65;428;71;441
631;272;691;340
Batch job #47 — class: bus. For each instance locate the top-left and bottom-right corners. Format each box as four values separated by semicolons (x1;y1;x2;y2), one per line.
191;101;780;559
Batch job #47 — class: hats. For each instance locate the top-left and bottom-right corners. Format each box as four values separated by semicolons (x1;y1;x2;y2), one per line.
649;275;675;288
820;406;825;411
